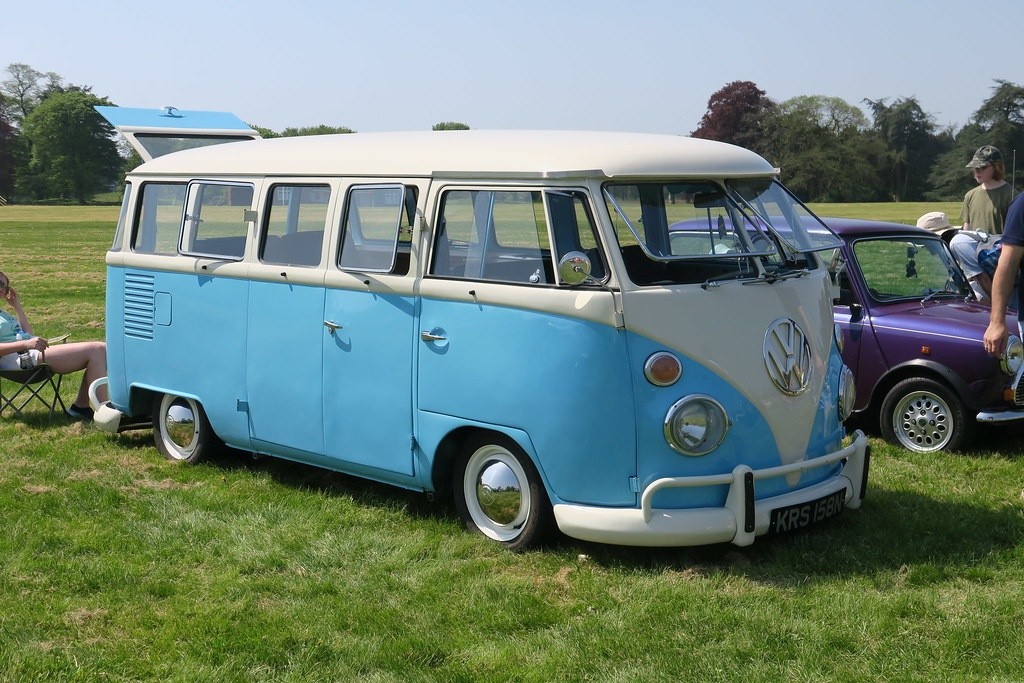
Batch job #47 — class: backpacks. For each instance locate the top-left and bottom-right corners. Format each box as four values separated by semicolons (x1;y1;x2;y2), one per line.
958;228;1022;284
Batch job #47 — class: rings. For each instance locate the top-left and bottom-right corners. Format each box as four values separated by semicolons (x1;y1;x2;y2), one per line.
984;346;988;348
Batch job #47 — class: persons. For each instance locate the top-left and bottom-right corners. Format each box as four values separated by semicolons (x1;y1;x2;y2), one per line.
983;188;1024;360
959;146;1019;235
907;212;1018;310
0;272;108;420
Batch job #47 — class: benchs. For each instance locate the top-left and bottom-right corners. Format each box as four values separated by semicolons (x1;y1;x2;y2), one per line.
270;231;364;269
192;235;290;263
449;257;555;284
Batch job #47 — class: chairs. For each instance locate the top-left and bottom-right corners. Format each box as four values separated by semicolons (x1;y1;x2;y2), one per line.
0;332;72;422
584;244;679;285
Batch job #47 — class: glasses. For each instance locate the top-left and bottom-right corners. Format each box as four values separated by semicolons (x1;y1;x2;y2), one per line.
0;280;10;294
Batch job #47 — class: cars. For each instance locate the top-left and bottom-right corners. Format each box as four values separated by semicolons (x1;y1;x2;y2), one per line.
669;218;1024;455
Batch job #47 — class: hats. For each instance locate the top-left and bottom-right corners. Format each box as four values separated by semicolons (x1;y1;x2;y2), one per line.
907;212;962;247
965;145;1002;168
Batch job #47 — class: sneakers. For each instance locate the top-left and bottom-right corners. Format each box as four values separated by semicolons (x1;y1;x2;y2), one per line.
90;402;127;420
66;403;94;421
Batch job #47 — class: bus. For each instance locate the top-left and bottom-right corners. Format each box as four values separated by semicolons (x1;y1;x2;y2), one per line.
87;105;871;553
